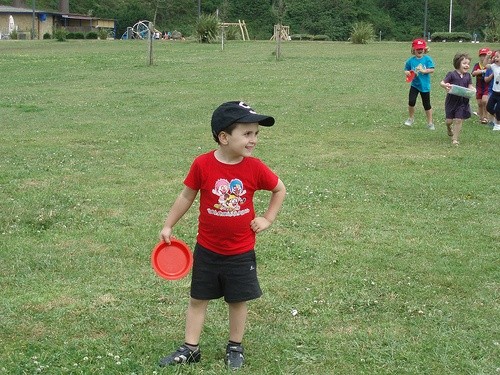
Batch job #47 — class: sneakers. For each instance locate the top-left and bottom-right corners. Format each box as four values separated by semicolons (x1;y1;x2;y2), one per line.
159;345;201;367
428;123;435;130
225;343;244;368
405;117;415;126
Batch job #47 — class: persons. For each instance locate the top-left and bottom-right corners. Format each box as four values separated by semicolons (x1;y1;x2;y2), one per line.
155;31;171;40
404;40;435;130
440;53;477;144
471;48;500;131
156;101;286;369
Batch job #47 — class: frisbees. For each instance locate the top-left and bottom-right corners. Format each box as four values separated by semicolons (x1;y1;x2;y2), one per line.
406;70;415;82
151;239;193;281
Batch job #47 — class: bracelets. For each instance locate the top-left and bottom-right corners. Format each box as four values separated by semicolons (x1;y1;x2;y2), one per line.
482;70;484;73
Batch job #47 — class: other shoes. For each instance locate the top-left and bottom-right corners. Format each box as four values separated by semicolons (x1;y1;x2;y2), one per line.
447;128;454;136
451;138;459;146
492;118;497;125
477;117;489;124
492;124;500;131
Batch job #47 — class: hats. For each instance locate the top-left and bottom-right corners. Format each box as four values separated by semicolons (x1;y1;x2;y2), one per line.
211;100;275;132
412;39;427;49
478;48;490;56
